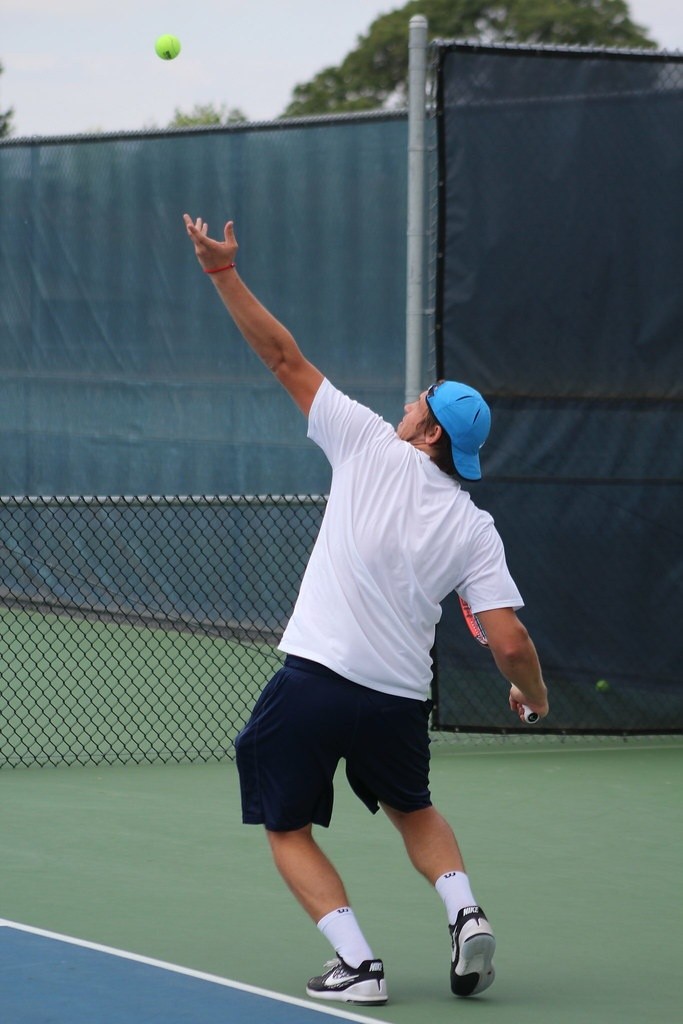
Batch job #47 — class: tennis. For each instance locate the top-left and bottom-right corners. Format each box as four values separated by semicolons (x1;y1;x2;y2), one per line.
596;680;609;693
156;35;181;60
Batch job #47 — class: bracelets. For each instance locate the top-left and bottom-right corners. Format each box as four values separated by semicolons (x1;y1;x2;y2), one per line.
203;264;236;273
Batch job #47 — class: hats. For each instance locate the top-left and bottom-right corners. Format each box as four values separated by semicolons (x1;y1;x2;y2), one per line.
426;379;491;481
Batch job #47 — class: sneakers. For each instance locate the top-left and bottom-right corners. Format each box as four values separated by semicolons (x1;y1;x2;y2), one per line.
448;906;495;997
306;950;388;1006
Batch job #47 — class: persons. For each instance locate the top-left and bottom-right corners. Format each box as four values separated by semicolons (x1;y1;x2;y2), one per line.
182;212;547;1004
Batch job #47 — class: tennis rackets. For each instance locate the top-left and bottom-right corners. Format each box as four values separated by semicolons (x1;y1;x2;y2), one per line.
458;591;539;724
